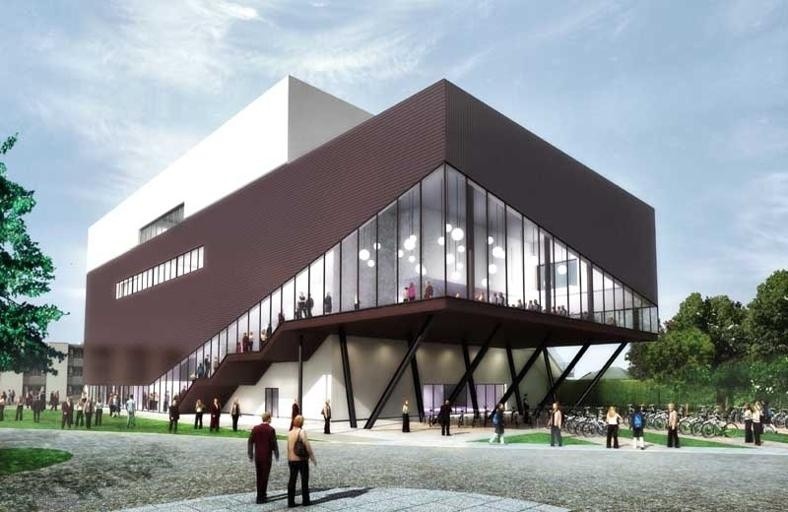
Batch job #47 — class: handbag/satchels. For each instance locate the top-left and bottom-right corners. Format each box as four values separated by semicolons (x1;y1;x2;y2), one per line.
293;430;309;457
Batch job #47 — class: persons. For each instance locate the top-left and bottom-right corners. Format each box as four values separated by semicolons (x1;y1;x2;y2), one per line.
667;403;680;448
437;400;452;436
547;401;562;447
742;400;777;446
0;385;331;507
605;406;622;448
488;403;505;445
403;280;615;327
629;406;645;450
195;292;332;378
523;393;530;417
402;400;410;432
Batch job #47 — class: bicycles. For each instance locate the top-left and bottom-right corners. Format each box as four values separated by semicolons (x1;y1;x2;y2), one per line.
455;409;465;429
481;406;489;428
427;409;435;428
542;404;787;439
508;406;521;429
